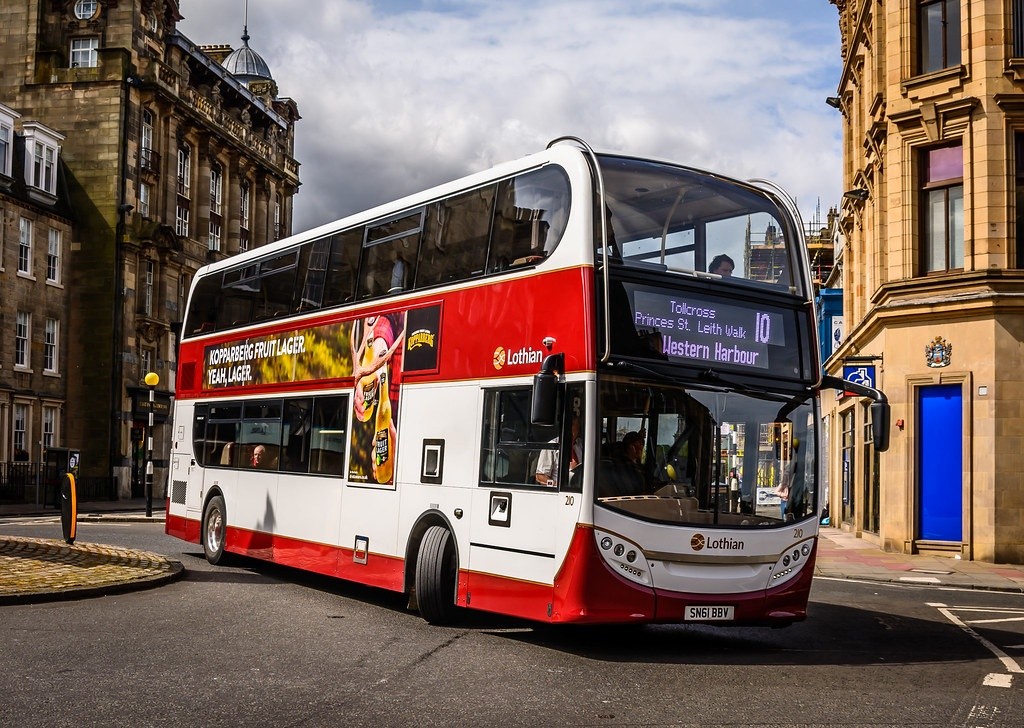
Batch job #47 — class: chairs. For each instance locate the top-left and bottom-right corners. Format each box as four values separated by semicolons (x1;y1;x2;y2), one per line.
223;442;276;469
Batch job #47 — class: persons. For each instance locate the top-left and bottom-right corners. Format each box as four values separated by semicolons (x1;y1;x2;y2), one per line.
708;254;734;276
534;415;583;487
248;445;265;467
772;474;788;518
610;430;645;496
731;474;739;513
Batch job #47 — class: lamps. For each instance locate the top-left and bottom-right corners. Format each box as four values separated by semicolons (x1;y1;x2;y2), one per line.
826;97;840;107
844;188;867;198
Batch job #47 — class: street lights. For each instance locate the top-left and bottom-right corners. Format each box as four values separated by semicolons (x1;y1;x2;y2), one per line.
144;373;159;516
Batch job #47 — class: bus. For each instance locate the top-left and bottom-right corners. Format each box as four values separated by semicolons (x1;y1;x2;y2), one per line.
164;135;891;634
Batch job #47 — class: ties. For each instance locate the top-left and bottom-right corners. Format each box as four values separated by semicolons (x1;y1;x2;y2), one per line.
571;443;579;466
